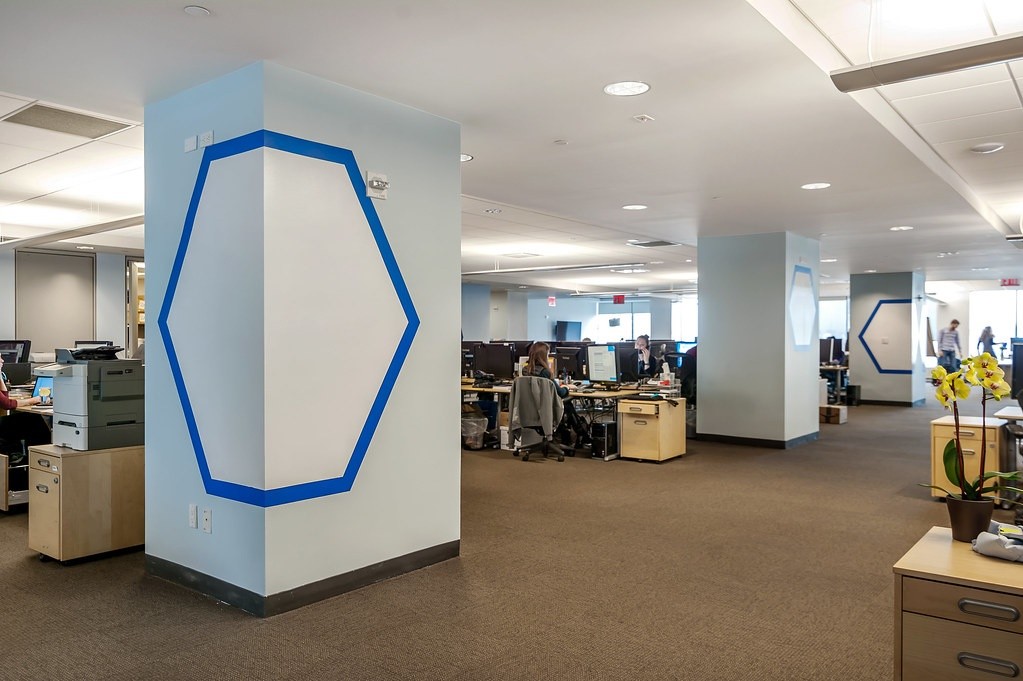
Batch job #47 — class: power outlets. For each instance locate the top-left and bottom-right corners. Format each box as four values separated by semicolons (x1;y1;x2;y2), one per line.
184;130;213;153
189;504;197;528
202;509;212;534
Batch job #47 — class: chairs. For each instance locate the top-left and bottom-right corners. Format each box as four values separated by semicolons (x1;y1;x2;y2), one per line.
512;376;576;461
664;354;696;409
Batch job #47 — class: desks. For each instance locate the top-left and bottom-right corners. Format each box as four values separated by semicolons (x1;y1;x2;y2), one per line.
820;365;848;404
993;406;1023;510
461;378;681;460
9;393;54;443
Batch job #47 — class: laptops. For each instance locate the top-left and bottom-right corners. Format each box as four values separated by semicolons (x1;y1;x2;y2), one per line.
31;376;54;401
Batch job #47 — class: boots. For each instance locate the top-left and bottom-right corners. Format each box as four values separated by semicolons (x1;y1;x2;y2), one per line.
569;408;588;445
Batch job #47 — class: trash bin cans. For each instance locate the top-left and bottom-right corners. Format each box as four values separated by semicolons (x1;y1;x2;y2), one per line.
461;412;488;449
845;385;861;406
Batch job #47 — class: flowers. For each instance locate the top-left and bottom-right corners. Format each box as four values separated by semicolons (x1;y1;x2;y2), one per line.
915;352;1023;505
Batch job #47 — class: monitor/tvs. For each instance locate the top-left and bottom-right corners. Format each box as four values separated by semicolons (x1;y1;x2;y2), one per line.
0;340;30;364
820;338;842;366
461;340;698;392
75;341;113;348
1010;338;1023;413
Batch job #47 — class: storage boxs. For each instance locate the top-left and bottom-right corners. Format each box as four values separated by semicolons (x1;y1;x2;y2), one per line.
500;425;521;452
820;405;847;424
500;412;510;426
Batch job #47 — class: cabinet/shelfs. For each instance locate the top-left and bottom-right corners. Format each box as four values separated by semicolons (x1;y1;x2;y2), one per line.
892;526;1023;681
616;396;686;462
930;415;1008;505
28;444;144;561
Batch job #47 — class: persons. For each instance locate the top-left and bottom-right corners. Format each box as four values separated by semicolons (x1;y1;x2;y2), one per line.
937;319;963;373
522;342;591;446
0;353;51;453
635;334;656;377
977;326;1002;358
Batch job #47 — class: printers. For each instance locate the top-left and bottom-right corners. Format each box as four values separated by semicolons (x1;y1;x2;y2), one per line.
34;346;145;452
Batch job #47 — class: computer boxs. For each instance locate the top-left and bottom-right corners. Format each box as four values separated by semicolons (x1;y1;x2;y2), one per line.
590;421;617;458
846;385;861;407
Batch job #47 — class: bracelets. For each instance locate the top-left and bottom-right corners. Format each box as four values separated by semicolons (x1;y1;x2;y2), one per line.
644;363;650;365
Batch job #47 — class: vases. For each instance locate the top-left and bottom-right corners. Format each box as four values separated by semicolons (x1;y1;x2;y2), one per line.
946;494;993;543
